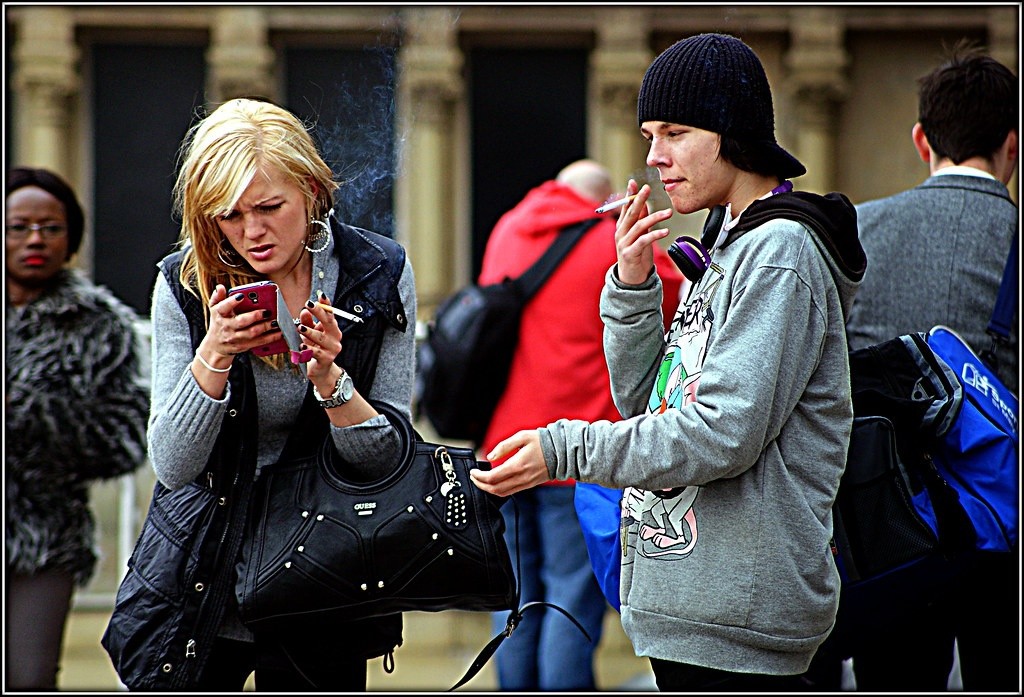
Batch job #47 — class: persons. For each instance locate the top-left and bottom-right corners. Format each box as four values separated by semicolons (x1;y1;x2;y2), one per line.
146;97;416;690
840;55;1019;692
5;165;152;692
471;33;868;691
478;159;683;690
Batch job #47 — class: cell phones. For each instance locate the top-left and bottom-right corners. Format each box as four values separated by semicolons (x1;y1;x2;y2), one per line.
228;283;291;357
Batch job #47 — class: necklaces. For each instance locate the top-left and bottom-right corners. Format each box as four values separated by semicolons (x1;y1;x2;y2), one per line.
293;319;301;324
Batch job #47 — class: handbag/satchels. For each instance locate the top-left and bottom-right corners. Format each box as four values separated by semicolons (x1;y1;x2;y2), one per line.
575;323;1019;660
408;275;526;447
229;397;593;692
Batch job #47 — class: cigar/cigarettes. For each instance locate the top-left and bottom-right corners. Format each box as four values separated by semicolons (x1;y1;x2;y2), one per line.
314;300;360;323
595;194;637;214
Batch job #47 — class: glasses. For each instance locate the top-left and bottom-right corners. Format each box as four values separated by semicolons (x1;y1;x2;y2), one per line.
7;223;68;240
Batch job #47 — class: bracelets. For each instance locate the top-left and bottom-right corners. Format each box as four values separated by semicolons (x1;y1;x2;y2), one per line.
196;348;232;373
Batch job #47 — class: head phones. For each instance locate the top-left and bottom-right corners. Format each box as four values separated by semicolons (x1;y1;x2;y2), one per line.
667;179;795;283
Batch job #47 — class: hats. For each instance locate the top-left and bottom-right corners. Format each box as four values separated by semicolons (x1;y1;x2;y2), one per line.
639;32;806;180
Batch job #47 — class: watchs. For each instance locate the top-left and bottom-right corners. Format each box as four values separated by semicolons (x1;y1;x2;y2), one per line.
313;367;354;409
